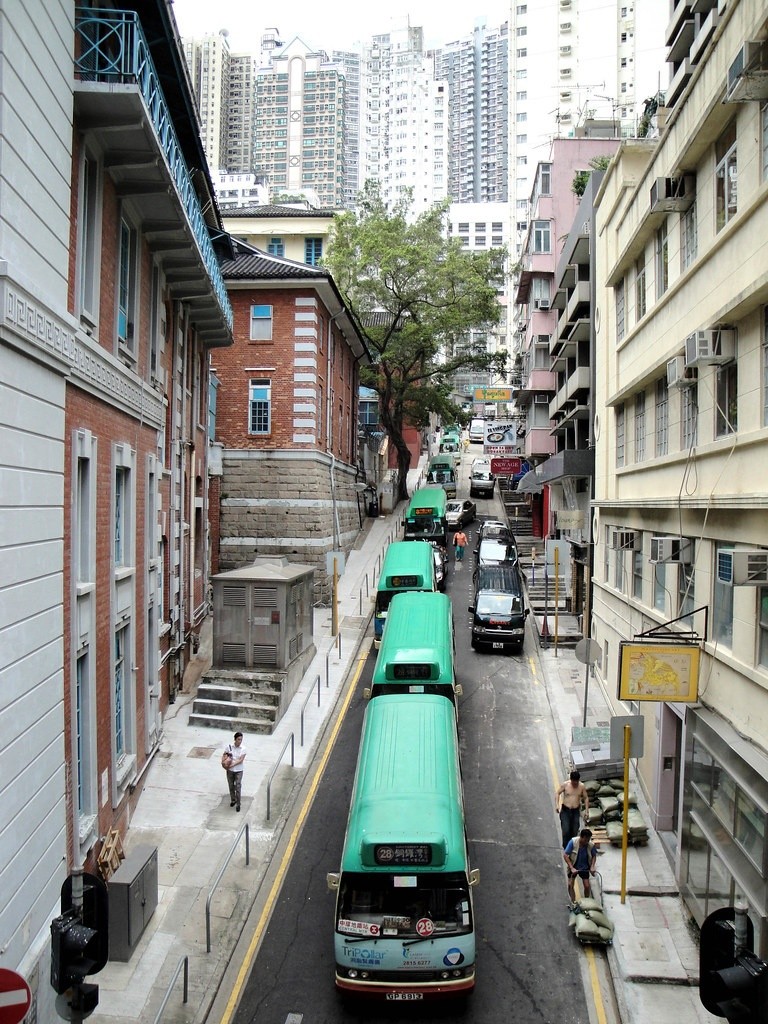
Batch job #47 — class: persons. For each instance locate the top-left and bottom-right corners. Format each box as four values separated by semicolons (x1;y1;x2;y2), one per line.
553;770;590;850
562;829;598;902
453;528;468;561
407;902;422;918
222;731;246;812
446;445;453;452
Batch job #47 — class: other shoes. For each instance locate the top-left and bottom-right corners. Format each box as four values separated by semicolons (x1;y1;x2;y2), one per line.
236;806;240;812
230;802;236;807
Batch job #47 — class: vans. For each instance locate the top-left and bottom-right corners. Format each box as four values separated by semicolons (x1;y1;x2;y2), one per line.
468;565;531;654
469;463;496;499
470;419;486;444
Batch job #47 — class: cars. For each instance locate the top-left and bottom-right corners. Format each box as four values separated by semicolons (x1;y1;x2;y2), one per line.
446;498;476;531
461;401;475;415
433;545;449;593
469;457;490;468
473;520;523;570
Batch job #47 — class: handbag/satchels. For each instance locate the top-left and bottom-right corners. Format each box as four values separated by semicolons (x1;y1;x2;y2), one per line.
453;534;457;546
221;745;233;770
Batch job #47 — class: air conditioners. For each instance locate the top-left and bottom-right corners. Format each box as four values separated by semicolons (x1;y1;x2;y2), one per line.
537;334;550;342
686;329;735;366
666;356;698;388
539;299;550;309
650;537;691;564
613;530;641;551
650;175;696;214
534;392;549;404
582;222;590;234
520;404;528;412
726;40;768;104
716;547;768;588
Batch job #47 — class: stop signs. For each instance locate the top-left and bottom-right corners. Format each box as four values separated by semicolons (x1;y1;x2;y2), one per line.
0;967;33;1024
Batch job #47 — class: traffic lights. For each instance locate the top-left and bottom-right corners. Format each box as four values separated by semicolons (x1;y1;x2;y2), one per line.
51;908;98;994
715;954;768;1024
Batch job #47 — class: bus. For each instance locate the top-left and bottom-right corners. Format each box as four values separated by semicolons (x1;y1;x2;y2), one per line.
401;487;448;547
370;541;441;650
363;590;463;722
424;455;458;498
439;422;462;465
327;695;482;1020
